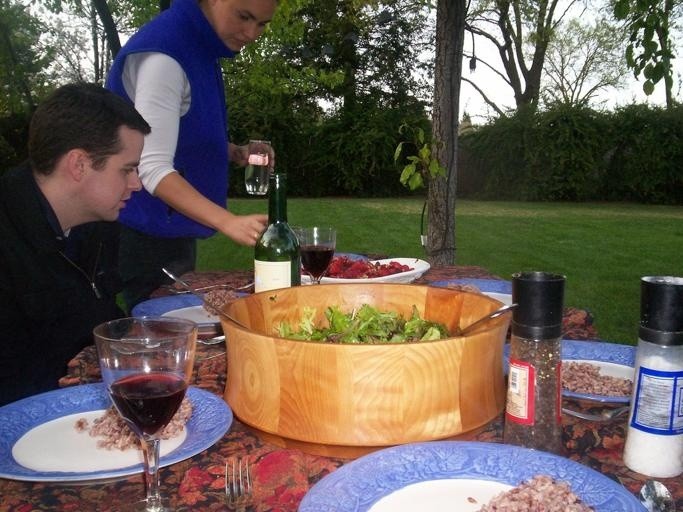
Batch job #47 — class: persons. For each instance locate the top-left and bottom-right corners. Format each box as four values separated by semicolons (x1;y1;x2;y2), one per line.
0;83;152;405
103;0;282;314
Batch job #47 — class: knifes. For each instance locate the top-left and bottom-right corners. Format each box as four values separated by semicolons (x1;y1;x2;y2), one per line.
157;267;249;328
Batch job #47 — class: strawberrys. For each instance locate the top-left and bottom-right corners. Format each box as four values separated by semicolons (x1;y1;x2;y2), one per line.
323;256;413;278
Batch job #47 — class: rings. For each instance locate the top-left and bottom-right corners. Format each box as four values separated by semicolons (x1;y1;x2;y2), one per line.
254;231;259;239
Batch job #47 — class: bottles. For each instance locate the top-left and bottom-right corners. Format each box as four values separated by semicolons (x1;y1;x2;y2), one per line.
621;272;682;474
498;269;568;457
253;172;299;294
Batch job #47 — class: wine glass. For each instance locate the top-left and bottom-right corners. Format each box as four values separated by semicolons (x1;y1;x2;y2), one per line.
89;311;202;508
296;224;336;283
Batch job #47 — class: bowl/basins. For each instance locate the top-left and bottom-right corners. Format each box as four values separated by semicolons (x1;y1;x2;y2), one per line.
215;282;511;444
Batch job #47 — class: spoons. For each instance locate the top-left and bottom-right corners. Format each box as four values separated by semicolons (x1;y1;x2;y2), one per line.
197;335;227;347
635;476;678;512
453;298;520;337
291;436;650;512
558;404;625;425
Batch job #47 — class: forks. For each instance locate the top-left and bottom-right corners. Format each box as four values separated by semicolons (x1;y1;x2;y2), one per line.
219;456;259;509
169;279;256;296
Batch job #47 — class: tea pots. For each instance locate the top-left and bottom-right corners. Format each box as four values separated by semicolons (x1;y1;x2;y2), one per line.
244;137;270;196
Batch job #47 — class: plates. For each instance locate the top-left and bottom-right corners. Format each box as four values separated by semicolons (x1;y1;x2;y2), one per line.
427;277;518;309
301;256;428;287
132;291;250;332
497;337;644;401
0;379;234;483
